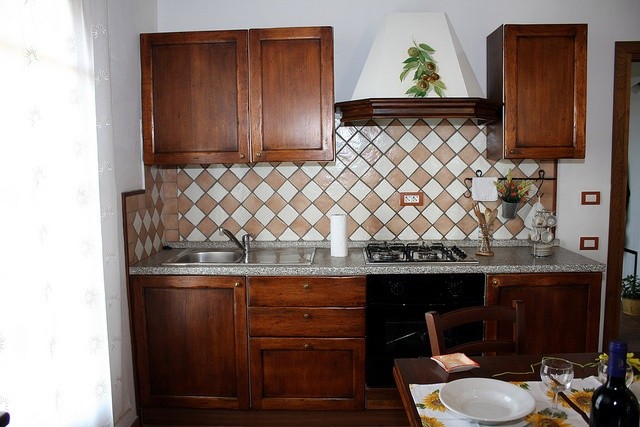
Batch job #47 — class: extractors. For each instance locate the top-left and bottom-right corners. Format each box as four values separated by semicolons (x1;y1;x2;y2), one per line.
337;12;487;119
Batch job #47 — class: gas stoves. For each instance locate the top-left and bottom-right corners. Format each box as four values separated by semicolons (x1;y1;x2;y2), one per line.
366;242;471;265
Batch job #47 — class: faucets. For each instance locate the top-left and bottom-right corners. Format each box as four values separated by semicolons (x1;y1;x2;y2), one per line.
218;226;244;253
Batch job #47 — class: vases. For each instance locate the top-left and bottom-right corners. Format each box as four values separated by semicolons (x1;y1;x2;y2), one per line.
500;203;519;219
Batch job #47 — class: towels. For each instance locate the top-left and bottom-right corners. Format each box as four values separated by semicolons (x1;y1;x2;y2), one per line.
562;375;640;419
409;381;588;427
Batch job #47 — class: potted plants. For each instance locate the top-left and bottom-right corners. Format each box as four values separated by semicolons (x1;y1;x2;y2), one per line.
619;274;640;316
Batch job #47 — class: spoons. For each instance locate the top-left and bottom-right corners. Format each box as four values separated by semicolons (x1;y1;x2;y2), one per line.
481;208;497;251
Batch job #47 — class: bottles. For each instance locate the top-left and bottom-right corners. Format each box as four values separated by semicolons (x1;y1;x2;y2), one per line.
590;340;640;427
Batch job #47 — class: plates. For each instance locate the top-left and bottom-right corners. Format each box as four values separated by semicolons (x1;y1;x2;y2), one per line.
438;377;537;426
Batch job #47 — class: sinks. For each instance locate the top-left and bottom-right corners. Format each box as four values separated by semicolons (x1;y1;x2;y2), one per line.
171;247;245;265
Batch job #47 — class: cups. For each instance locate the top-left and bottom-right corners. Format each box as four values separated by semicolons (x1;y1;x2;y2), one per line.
528;209;558;243
598;360;633;387
478;224;494;256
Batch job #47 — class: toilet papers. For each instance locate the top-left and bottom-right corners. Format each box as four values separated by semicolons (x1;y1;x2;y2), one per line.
330;214;347;257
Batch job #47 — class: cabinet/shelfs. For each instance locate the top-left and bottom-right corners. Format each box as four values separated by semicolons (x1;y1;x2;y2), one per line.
246;276;366;412
485;23;588;159
484;273;602;356
127;272;250;415
140;28;336;167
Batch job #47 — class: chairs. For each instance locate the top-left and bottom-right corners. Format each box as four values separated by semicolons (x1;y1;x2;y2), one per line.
420;300;525;354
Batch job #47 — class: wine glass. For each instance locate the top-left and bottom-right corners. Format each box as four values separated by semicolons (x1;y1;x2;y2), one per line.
540;357;577;418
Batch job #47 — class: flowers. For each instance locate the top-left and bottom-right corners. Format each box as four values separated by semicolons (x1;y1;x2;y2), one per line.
494;178;532;203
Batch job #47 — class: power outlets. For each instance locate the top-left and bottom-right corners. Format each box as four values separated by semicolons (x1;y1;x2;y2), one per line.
400;193;424;206
